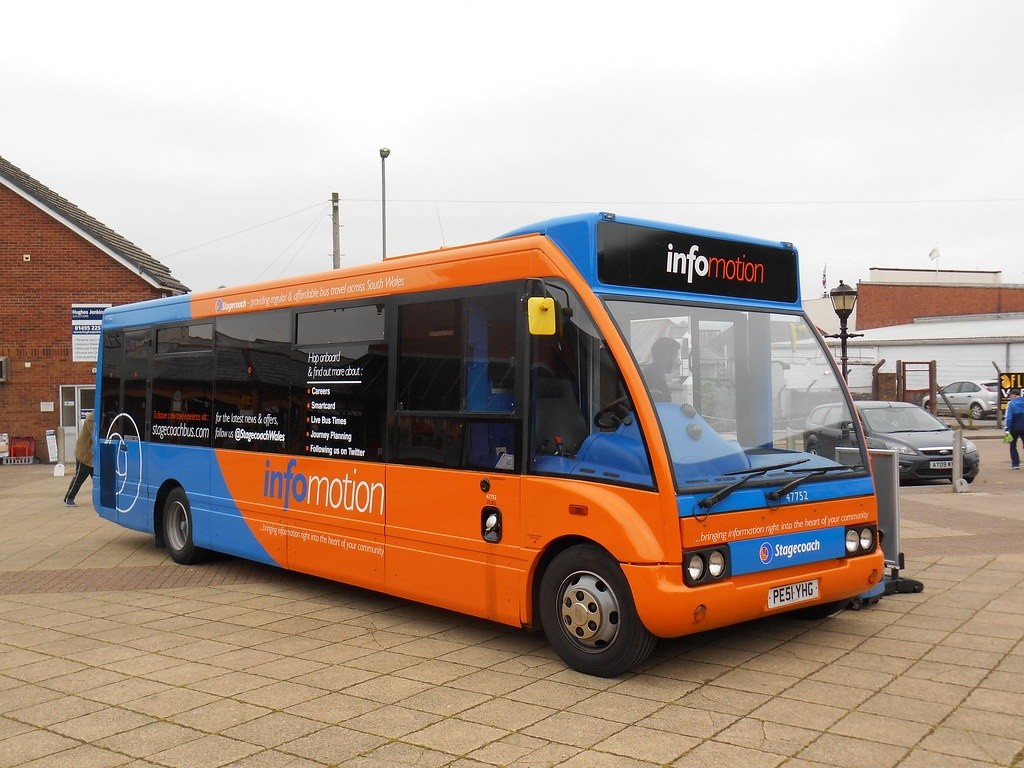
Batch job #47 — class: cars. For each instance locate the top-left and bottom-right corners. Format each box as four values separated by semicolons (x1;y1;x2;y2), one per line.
802;399;980;486
922;379;999;420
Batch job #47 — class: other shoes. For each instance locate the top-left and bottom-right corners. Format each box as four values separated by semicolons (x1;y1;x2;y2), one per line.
64;500;78;507
1009;465;1019;470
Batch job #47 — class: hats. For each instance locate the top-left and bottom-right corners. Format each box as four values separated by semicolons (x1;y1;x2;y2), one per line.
1007;389;1020;396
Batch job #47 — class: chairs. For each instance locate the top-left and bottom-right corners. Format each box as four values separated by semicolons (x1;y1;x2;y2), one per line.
524;364;588;459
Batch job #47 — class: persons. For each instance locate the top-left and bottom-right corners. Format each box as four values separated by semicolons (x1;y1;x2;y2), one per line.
642;337;682;402
63;409;95;508
1004;389;1024;470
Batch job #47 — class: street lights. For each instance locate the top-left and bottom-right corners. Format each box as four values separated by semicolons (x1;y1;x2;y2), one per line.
829;280;864;450
381;148;390;257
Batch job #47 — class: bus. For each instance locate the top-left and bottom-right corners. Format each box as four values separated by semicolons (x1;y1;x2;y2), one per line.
92;210;887;679
